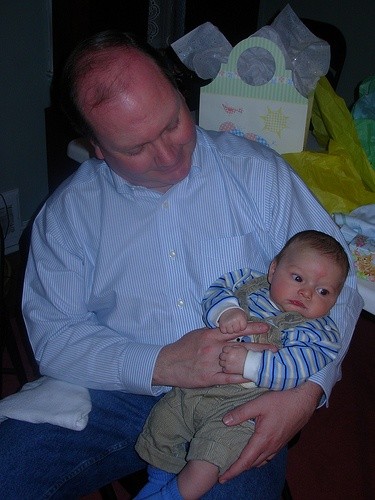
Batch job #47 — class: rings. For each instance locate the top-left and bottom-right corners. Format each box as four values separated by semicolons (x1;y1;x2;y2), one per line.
266;458;271;463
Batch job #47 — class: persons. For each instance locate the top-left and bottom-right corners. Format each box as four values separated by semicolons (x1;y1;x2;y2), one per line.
0;46;366;499
130;229;350;500
